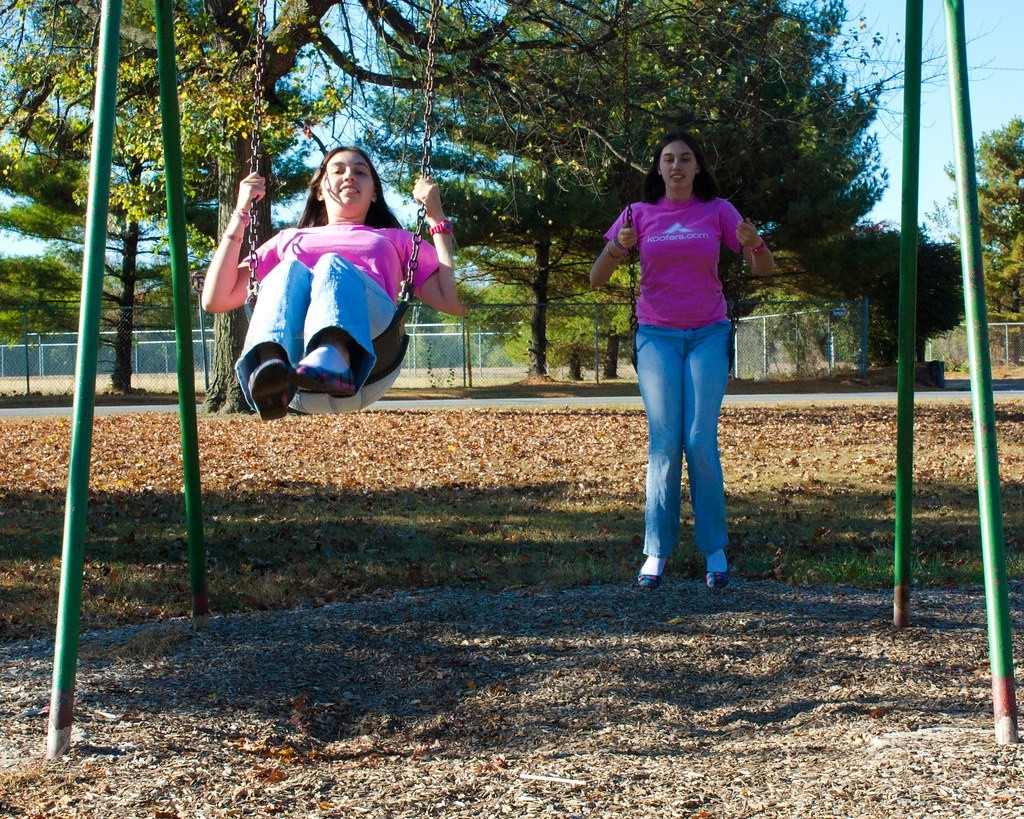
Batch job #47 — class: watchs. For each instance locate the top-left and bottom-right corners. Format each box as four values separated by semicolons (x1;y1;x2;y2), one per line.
430;220;453;236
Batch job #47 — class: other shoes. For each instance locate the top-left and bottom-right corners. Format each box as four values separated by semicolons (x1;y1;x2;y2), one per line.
248;359;289;420
637;564;666;588
294;365;356;397
707;562;730;590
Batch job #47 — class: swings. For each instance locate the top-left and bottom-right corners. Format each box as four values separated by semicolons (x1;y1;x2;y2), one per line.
243;0;446;419
620;0;773;375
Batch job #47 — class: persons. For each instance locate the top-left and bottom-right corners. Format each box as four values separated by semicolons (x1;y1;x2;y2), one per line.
201;145;466;420
589;133;774;588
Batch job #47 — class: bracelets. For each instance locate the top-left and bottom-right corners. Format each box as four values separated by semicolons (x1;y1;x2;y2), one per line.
232;210;249;226
608;239;624;258
748;237;766;254
222;233;243;243
